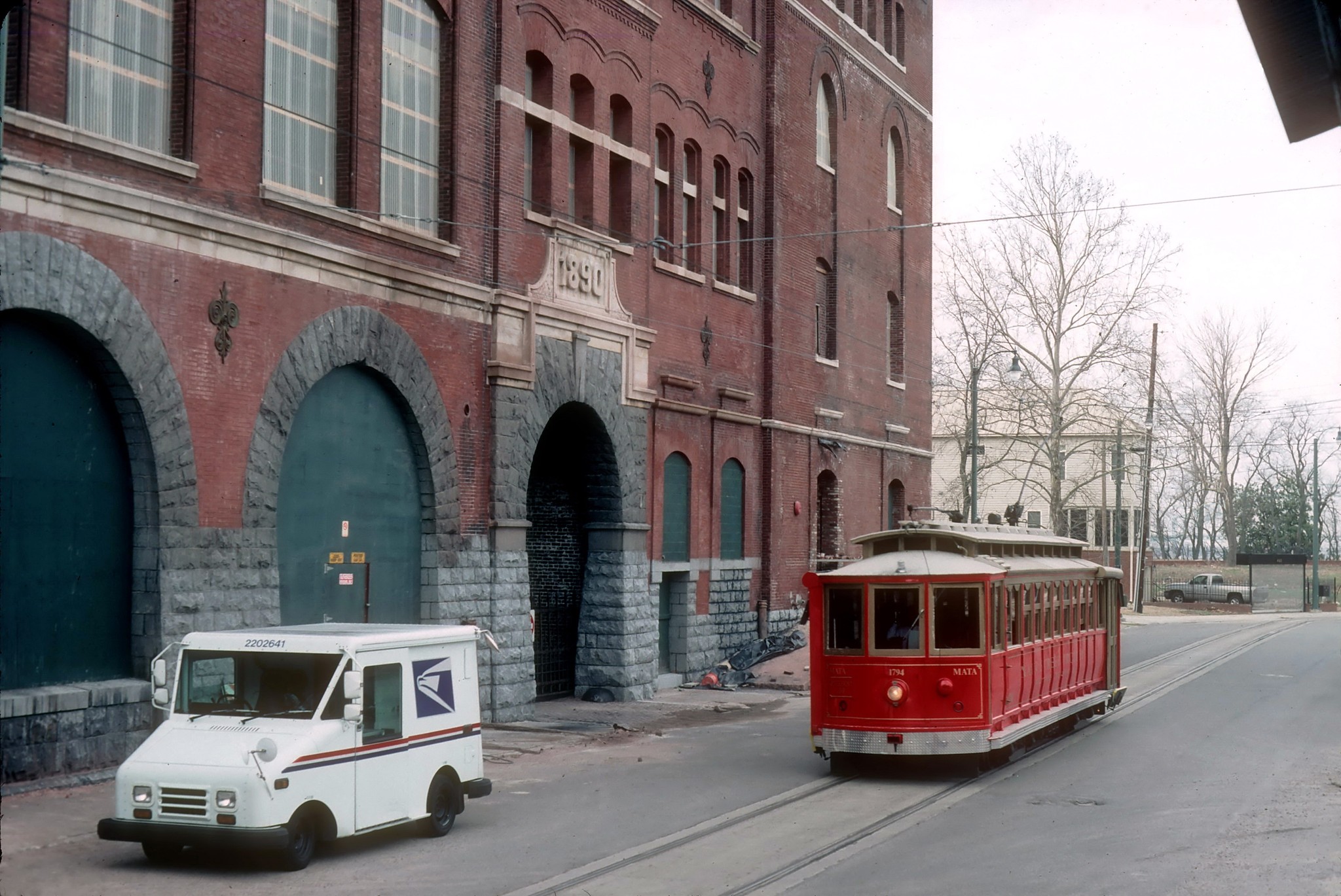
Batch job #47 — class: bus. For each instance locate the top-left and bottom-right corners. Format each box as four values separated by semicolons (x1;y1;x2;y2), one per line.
798;505;1128;775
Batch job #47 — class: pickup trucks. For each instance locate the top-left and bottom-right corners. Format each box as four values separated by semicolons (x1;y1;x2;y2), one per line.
1163;573;1269;605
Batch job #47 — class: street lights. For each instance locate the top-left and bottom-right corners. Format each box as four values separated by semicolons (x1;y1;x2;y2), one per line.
1113;407;1154;567
1313;426;1341;607
971;349;1023;522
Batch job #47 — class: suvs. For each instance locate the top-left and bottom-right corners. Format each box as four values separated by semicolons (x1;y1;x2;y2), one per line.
98;622;494;871
